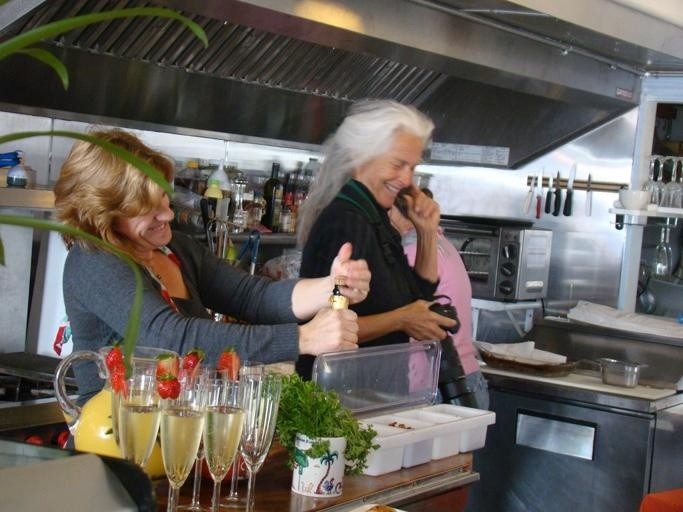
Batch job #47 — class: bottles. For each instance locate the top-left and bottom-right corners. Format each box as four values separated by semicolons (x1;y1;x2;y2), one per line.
174;155;320;235
298;275;352;380
6;150;28;187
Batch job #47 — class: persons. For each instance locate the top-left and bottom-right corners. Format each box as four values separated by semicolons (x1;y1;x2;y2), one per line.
387;184;492;411
52;124;372;449
294;97;456;383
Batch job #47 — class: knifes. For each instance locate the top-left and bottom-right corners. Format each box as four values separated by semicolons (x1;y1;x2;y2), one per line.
521;163;592;220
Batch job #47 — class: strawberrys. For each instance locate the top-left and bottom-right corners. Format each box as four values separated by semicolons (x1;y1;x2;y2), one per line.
157;373;181;399
217;347;239;381
112;367;126;393
106;347;123;370
182;347;204;376
156;353;179;380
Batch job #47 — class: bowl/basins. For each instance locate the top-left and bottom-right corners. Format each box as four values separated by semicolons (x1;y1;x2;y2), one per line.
595;356;651;390
617;188;651;209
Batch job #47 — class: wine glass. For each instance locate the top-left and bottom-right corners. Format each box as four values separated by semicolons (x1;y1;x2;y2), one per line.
115;357;283;512
647;152;683;280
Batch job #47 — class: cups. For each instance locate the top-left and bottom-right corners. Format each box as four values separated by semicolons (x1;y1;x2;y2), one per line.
290;424;347;501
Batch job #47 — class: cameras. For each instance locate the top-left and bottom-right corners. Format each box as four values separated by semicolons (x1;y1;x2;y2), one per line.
421;301;478;411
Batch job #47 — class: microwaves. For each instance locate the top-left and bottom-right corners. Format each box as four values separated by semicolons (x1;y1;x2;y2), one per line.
436;218;553;302
